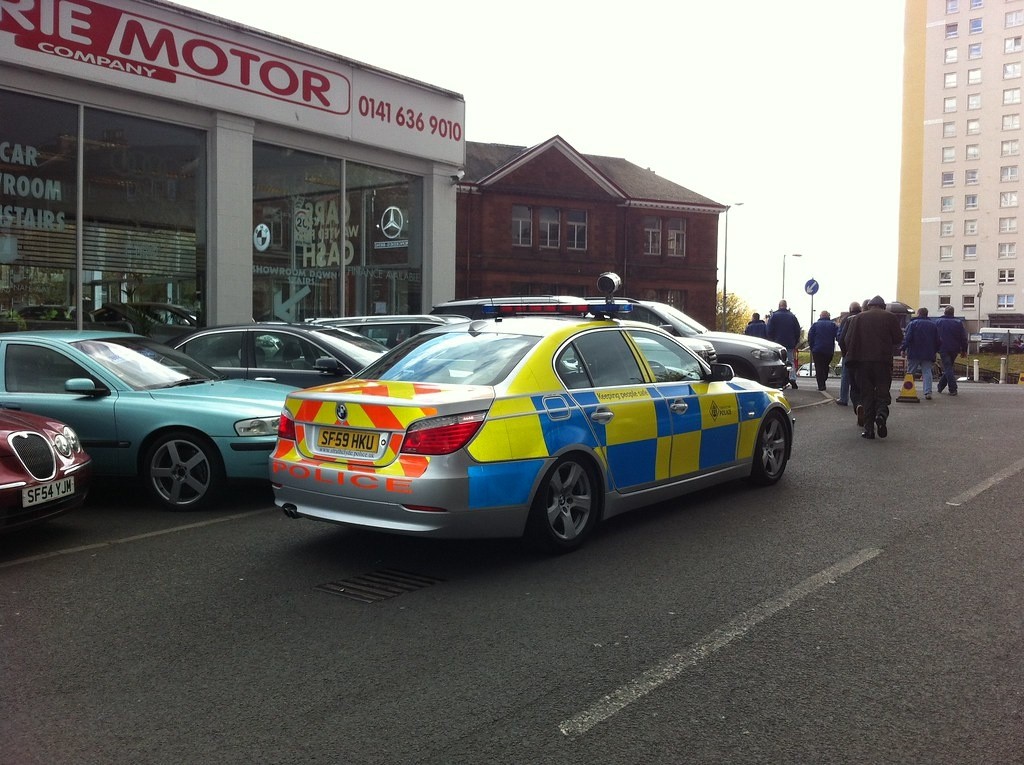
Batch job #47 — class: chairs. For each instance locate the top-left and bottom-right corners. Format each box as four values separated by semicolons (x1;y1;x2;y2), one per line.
256;347;275;368
282;342;312;369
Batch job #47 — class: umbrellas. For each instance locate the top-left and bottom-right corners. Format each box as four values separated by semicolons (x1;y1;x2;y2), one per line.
884;301;914;314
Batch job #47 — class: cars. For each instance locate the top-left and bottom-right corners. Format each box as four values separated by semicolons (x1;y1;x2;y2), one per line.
796;362;838;377
11;303;100;322
0;328;305;511
265;296;798;556
141;320;398;389
88;301;197;331
0;407;96;540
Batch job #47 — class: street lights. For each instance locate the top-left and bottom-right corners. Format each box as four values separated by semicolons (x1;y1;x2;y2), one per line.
722;202;745;332
782;253;803;299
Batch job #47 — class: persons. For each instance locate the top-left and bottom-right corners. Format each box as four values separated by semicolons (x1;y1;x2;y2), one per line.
807;311;838;391
844;295;903;439
837;299;871;424
901;307;939;399
936;305;968;396
745;313;767;338
765;300;801;389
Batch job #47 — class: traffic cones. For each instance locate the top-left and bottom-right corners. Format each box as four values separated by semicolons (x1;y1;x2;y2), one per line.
1018;373;1024;385
895;371;920;403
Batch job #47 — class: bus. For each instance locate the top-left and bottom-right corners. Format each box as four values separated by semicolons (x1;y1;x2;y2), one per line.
979;326;1024;354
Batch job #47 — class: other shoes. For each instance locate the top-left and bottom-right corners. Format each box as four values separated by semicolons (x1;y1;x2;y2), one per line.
817;386;826;391
876;414;887;438
835;399;848;406
948;390;957;395
856;405;866;427
789;379;798;389
937;384;942;393
861;431;876;439
925;394;932;400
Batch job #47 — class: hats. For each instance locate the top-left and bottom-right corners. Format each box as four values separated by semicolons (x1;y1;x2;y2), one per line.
867;296;887;310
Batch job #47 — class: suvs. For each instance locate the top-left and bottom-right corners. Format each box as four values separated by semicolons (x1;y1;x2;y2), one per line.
300;291;797;392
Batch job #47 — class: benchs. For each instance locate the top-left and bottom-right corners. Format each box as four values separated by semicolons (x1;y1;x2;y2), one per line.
195;344;241;367
